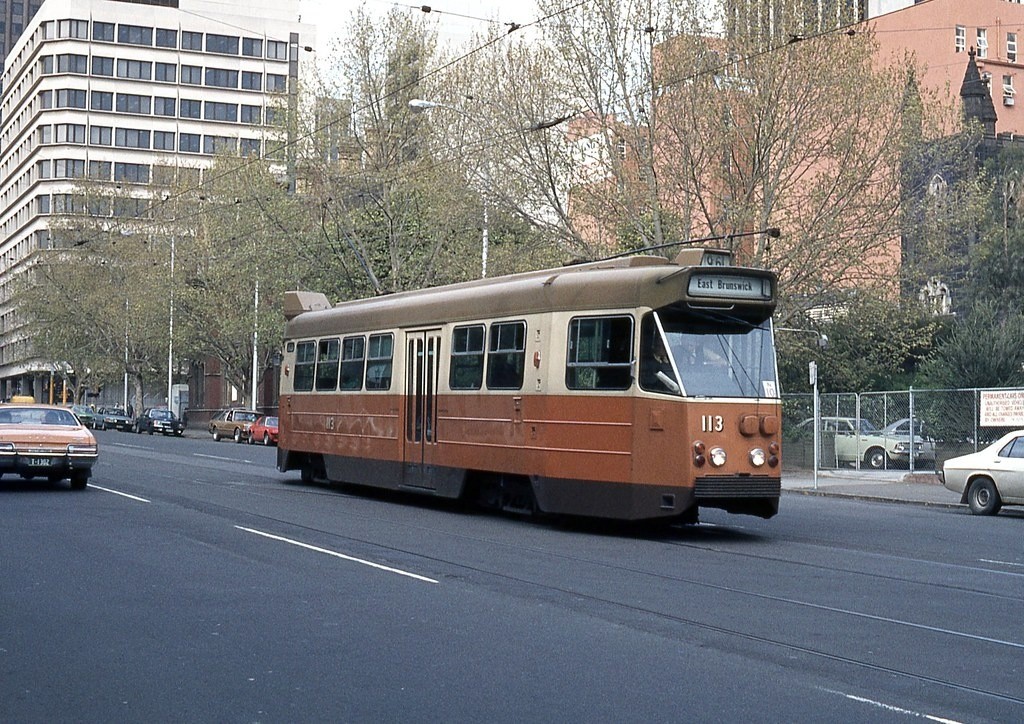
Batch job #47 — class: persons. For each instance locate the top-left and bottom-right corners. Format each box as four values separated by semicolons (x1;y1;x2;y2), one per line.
181;408;190;431
647;327;731;375
115;401;133;418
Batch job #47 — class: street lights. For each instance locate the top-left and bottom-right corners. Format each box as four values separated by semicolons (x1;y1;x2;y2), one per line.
408;98;488;280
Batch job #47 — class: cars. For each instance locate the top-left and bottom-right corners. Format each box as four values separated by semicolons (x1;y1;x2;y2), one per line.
71;404;96;426
790;418;922;470
0;395;98;493
208;409;265;444
96;406;134;432
933;430;1024;516
880;417;988;463
247;415;279;447
135;408;185;437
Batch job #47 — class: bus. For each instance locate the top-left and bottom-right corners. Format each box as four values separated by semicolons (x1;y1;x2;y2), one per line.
275;242;785;531
